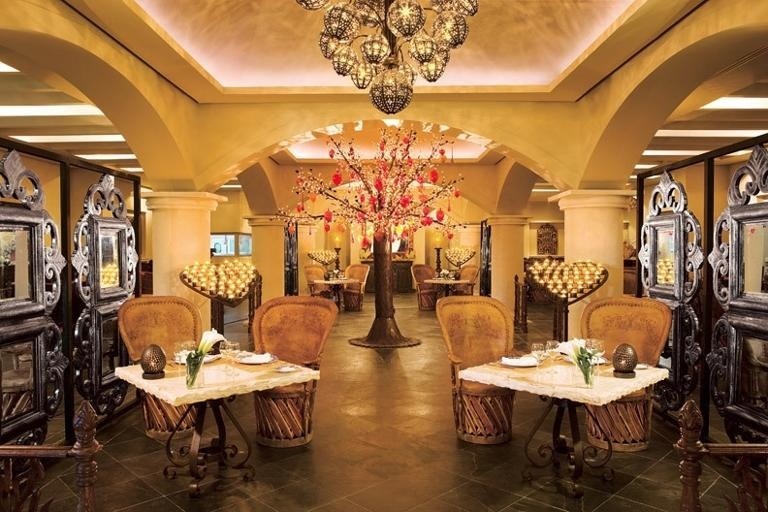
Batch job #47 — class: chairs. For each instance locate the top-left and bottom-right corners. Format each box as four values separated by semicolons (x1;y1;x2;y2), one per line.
411;262;436;311
436;295;519;444
302;263;327;301
117;296;202;439
459;264;479;296
580;297;673;453
343;263;371;310
252;296;339;448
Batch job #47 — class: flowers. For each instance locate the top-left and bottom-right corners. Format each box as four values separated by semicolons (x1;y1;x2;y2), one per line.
570;334;599;385
187;329;223;384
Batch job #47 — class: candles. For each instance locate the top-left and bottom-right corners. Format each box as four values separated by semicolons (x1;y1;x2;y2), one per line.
526;253;606;302
447;245;476;267
182;255;260;305
434;237;444;275
307;247;338;264
334;235;341;249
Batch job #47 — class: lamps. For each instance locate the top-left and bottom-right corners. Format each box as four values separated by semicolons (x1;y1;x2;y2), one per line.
295;1;479;114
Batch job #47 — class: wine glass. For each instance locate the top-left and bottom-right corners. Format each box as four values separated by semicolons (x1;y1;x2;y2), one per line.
433;269;456;282
171;336;241;383
532;338;606;377
328;269;348;280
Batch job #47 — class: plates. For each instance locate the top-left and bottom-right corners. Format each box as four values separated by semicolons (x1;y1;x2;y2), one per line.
238;355;278;364
273;365;300;372
498;356;538;368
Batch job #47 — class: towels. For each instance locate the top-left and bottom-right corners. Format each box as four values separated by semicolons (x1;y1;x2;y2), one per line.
501;356;538;366
241;353;274;363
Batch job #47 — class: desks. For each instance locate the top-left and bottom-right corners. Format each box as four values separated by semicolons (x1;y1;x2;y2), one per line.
424;274;470;299
314;279;359;300
